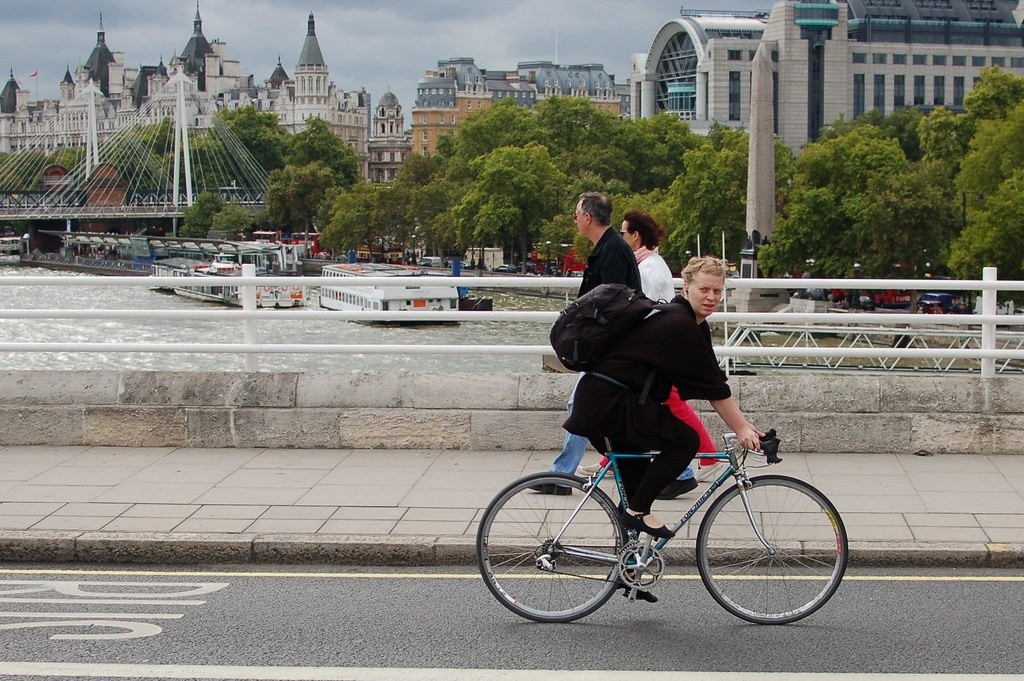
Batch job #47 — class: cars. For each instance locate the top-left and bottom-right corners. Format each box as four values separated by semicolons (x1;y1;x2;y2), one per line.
916;300;945;315
493;263;518;274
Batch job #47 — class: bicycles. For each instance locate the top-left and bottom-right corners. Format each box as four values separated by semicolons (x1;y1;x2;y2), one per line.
476;427;850;626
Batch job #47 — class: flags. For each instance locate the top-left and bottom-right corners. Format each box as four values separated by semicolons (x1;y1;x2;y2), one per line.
30;71;38;77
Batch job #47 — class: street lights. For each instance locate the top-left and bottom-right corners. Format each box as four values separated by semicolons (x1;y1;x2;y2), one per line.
545;239;552;275
410;233;417;266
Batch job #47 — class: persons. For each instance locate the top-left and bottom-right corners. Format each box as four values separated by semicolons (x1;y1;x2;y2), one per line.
563;257;766;602
529;191;699;500
578;211;724;480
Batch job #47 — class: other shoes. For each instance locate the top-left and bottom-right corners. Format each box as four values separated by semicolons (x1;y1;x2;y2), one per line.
577;463;614;479
623;587;658;603
620;510;675;539
654;476;699;501
528;483;572;495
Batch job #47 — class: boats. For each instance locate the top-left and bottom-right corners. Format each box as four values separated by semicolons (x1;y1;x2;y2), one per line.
144;251;304;308
317;262;460;326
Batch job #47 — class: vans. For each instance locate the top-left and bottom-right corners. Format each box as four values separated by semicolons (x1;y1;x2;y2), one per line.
918;292;954;313
417;256;442;267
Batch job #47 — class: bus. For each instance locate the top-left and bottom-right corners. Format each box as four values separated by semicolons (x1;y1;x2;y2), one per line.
252;228;292;254
790;258;913;309
290;231;333;260
529;242;586;277
356;234;404;265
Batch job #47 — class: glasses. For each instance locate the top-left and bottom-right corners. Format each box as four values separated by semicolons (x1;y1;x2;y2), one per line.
620;229;628;237
573;214;584;220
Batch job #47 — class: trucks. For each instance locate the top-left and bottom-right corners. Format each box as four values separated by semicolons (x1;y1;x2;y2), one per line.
463;247;504;271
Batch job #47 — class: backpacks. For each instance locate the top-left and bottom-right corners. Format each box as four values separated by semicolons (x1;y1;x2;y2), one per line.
551;284;688;373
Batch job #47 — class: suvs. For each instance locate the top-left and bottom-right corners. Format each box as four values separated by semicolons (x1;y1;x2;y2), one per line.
517;261;532;272
